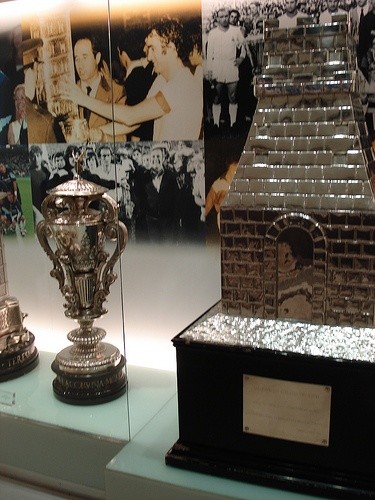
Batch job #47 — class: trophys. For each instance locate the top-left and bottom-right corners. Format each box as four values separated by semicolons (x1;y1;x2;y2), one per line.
164;14;375;500
37;175;129;406
0;242;40;383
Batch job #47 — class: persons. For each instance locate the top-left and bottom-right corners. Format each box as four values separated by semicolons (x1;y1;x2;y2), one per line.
1;0;375;241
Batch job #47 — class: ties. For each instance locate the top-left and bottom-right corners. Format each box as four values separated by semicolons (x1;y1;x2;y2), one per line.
359;9;364;21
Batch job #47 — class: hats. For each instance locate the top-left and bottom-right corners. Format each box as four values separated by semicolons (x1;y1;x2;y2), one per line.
21;38;46;69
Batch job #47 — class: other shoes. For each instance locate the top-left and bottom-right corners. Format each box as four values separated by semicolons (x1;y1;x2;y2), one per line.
229;122;242;134
211;124;220;135
